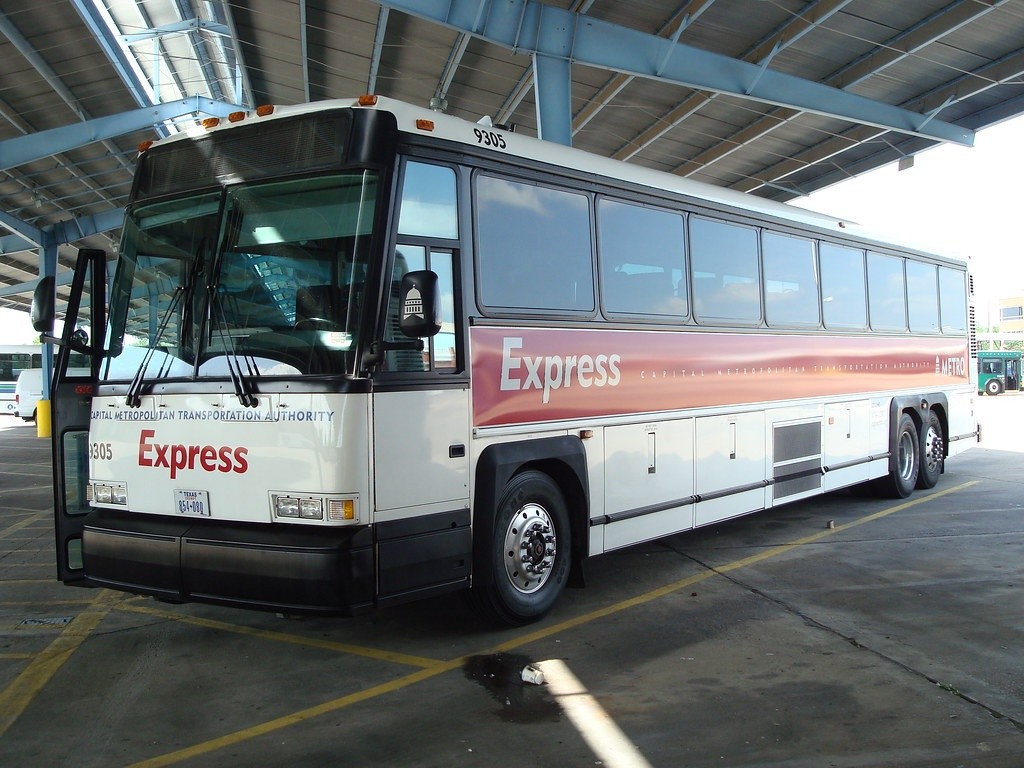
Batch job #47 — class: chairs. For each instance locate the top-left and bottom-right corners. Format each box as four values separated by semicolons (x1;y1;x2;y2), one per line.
294;284;341;332
600;271;838;328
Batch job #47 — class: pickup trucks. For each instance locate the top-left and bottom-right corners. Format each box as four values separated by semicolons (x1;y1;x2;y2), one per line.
14;366;90;427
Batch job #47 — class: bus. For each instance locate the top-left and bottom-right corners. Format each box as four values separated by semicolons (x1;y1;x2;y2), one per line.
977;350;1024;396
30;91;983;626
0;341;178;416
